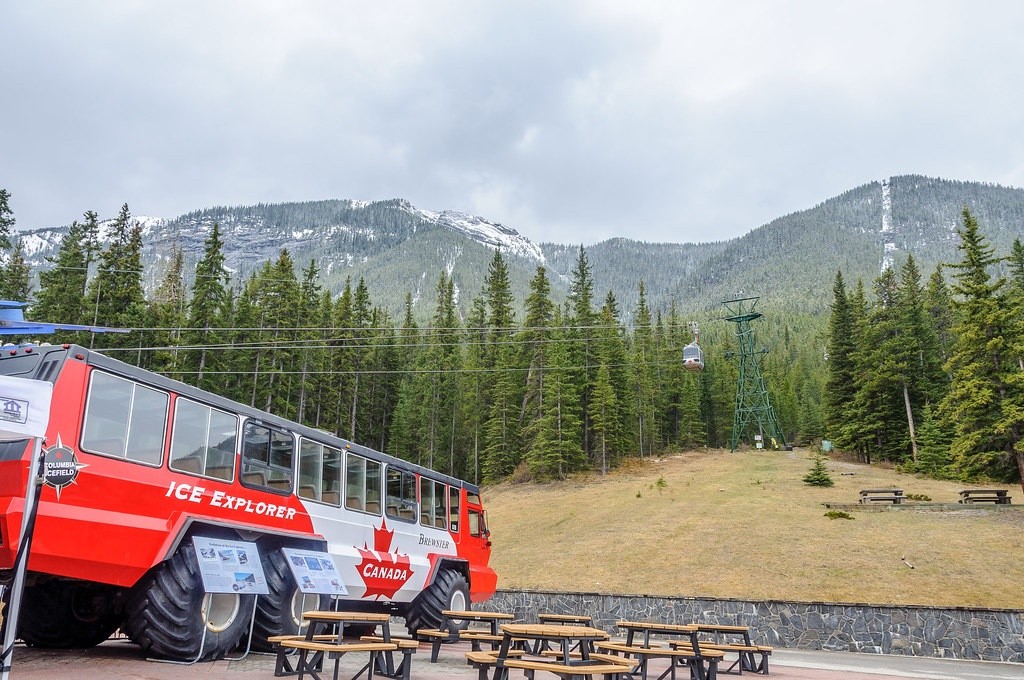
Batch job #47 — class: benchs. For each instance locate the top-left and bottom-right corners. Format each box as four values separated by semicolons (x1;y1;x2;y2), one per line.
958;496;1012;505
858;496;907;505
268;629;774;680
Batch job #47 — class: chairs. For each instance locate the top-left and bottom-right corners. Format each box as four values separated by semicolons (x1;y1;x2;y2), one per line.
88;438;447;528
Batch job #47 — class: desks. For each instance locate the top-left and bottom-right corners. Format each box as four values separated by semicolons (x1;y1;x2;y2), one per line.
959;489;1009;504
299;610;394;675
433;612;515;661
686;623;756;671
492;623;606;680
859;489;904;505
617;622;707;680
535;614;594;654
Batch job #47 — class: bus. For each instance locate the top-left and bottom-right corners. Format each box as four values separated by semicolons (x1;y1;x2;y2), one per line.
0;337;497;667
